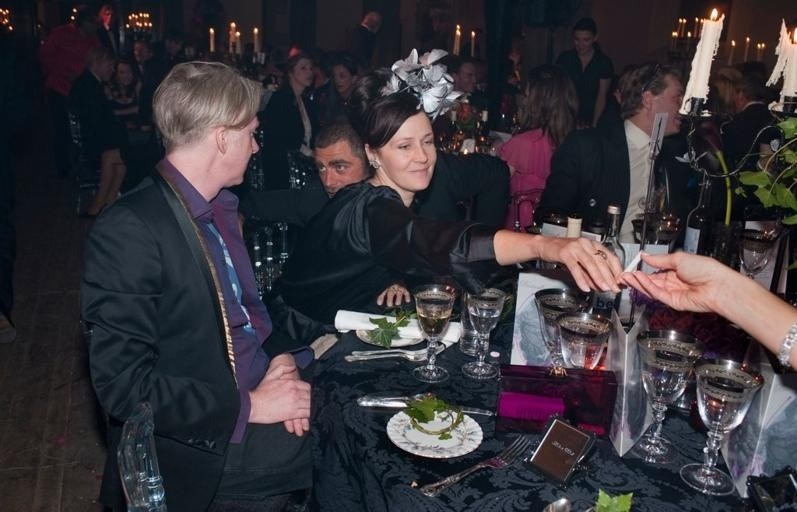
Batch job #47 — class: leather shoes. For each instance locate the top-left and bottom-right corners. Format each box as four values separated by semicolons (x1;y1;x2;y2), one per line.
0;310;17;343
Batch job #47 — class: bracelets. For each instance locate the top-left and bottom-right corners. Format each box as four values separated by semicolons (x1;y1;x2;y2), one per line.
775;308;797;368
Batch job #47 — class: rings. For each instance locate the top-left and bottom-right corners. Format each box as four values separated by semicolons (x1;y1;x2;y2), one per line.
589;247;607;265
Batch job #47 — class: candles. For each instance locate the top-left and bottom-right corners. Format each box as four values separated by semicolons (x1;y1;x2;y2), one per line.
0;11;10;26
679;13;726;116
229;31;233;53
230;26;236;42
456;35;460;55
210;33;214;51
235;36;240;54
126;16;152;30
472;36;475;56
764;17;797;112
253;33;258;52
453;30;459;55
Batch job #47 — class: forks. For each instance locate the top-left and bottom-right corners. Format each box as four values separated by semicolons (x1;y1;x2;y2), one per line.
343;345;445;361
419;434;531;500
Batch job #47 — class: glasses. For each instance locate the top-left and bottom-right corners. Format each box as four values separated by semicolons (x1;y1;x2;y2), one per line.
642;64;661;90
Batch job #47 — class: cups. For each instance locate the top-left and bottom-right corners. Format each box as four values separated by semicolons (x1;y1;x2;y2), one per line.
459;293;491;357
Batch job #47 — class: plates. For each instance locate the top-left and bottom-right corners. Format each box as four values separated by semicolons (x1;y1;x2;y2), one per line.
386;407;484;459
356;331;427;347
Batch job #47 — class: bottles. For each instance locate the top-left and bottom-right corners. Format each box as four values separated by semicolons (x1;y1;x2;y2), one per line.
556;212;582;271
684;174;717;257
590;205;626;314
476;110;489;135
449;109;457;136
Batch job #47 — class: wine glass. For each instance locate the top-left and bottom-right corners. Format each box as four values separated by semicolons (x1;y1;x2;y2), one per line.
680;364;765;495
739;229;775;284
632;338;701;463
411;283;456;384
656;212;682;243
534;289;593;376
462;287;507;380
553;311;613;371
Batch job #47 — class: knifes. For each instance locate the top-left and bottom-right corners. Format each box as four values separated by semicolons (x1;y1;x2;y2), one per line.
356;394;495;418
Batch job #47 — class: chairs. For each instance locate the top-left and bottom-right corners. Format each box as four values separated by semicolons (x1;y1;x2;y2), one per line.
513;187;545;232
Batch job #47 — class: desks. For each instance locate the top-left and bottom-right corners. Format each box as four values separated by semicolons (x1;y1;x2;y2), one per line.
322;260;797;511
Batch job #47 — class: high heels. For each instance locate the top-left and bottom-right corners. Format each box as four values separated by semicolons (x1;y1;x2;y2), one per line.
76;202;106;218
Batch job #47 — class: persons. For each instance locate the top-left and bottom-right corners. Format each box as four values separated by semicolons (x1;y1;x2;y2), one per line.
38;22;77;157
103;58;162;197
131;39;166;133
284;42;326;87
241;114;484;228
312;68;328;87
498;65;579;230
316;57;361;116
457;152;520;284
172;44;281;93
88;3;121;60
262;47;622;355
342;10;381;74
266;54;319;158
534;59;698;228
79;61;313;511
704;81;733;113
446;55;498;130
72;50;126;217
597;63;639;126
499;60;523;116
559;19;624;129
722;61;780;221
2;170;18;343
623;251;796;368
